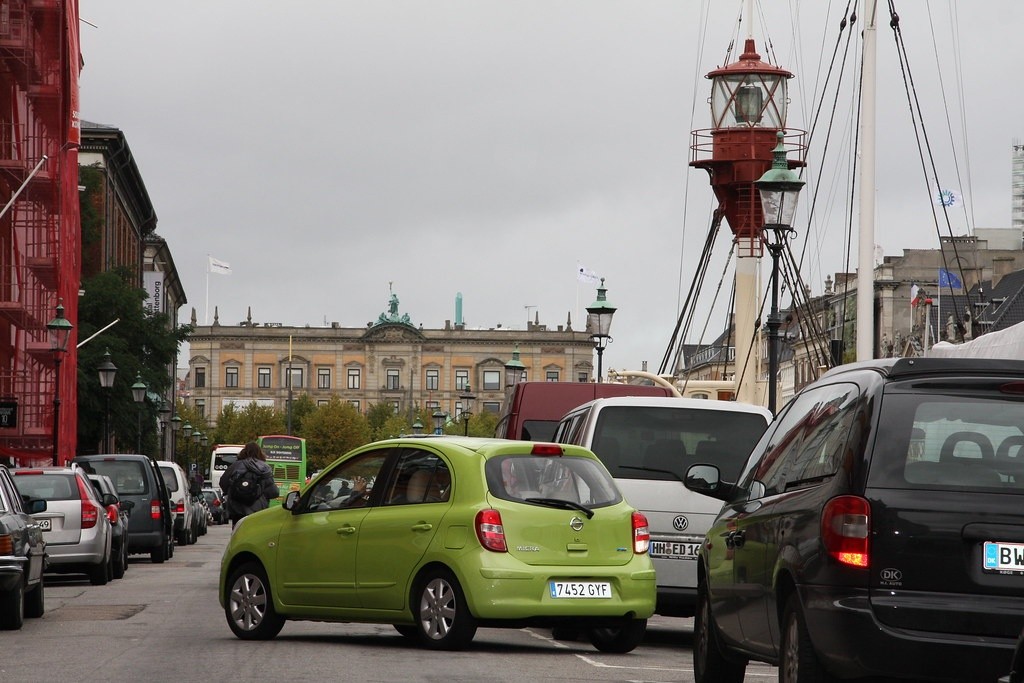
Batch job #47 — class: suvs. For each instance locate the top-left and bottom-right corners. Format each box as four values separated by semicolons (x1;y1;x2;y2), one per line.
9;463;119;587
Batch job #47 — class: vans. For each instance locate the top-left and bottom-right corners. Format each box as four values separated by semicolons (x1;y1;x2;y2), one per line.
72;455;173;563
494;380;673;443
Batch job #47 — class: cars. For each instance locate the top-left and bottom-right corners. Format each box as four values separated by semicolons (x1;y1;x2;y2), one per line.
0;464;48;631
87;475;135;579
683;355;1024;683
218;436;658;655
149;460;227;546
552;396;776;618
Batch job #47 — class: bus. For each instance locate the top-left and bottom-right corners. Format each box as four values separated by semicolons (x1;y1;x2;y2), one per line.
257;435;306;508
210;444;246;493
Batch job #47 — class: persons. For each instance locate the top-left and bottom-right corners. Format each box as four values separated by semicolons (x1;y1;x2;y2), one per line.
218;442;279;533
881;333;888;359
341;477;368;507
892;328;902;357
946;311;956;341
963;306;973;338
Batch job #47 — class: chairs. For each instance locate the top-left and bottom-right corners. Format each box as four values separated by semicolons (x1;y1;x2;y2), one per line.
643;441;683;472
406;468;442;503
595;434;622;469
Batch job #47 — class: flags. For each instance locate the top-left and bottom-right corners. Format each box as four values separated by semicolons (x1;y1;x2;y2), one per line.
939;267;962;289
933;180;964;212
910;280;924;308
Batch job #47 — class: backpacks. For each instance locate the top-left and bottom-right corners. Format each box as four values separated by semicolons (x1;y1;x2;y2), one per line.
231;470;265;507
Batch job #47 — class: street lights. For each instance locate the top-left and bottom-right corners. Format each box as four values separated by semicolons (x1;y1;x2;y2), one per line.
170;409;182;463
459;380;477;435
157;397;171;461
585;276;618;383
191;425;201;474
200;429;209;480
183;419;193;479
96;347;118;455
752;131;807;418
131;371;147;455
45;297;74;466
